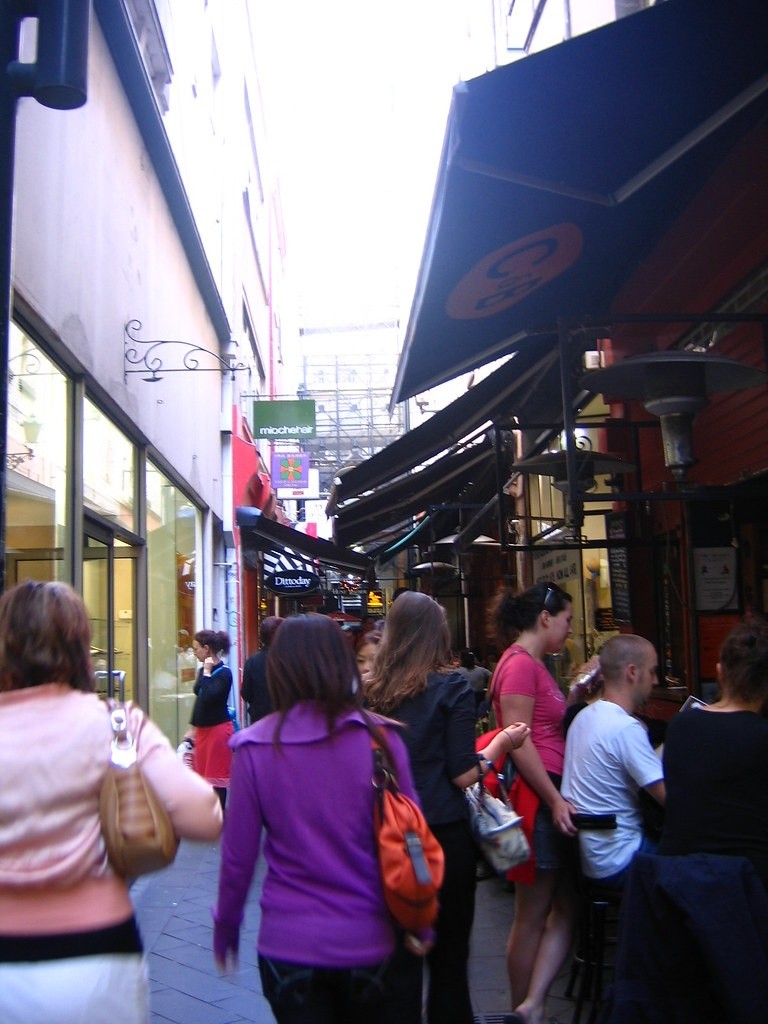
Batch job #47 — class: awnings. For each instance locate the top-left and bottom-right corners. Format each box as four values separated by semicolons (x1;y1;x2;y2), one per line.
387;1;768;414
332;425;496;550
379;511;437;566
251;514;378;585
325;344;549;518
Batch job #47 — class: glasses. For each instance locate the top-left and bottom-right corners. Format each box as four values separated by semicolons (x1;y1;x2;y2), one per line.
542;582;557;608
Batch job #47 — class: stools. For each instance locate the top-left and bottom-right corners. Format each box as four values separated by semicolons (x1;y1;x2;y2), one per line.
541;806;625;1024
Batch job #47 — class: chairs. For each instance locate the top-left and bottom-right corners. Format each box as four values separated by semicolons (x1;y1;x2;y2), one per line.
596;852;768;1024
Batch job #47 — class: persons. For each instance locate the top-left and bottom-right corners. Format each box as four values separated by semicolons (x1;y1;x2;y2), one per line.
0;580;589;1023
655;609;768;895
559;634;666;894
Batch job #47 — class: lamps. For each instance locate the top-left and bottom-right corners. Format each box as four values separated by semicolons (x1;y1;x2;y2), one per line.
0;0;89;110
577;349;768;495
511;450;637;545
5;411;45;470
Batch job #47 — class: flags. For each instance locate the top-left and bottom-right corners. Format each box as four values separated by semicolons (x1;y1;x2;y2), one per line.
288;521;319;572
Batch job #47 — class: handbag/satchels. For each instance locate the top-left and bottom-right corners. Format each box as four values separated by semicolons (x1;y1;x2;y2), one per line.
370;725;443;934
465;757;530;875
100;697;181;875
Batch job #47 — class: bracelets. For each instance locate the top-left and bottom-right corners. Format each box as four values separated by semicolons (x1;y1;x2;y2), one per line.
502;730;515;749
